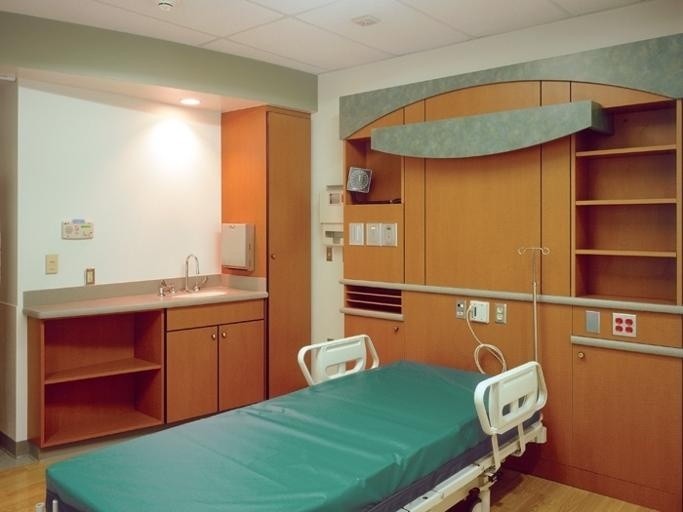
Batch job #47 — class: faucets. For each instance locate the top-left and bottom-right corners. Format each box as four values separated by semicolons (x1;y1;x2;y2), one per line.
184;253;200;290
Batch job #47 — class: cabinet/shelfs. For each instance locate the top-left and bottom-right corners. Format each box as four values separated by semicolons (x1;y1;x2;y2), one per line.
220;105;311;401
340;35;682;511
26;299;269;460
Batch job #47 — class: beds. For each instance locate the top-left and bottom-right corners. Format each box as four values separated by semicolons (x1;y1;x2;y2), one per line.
44;359;547;512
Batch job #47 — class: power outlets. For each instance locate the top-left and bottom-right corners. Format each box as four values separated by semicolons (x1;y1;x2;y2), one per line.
44;253;58;274
85;267;96;285
468;300;491;325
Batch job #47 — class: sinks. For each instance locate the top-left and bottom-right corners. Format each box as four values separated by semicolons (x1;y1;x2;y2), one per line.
172;290;227;297
